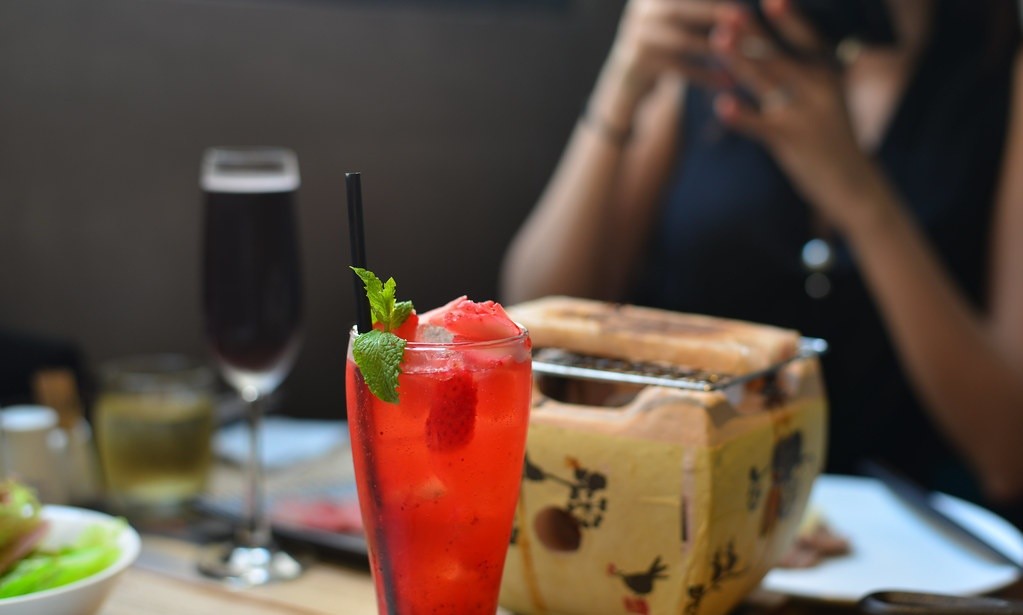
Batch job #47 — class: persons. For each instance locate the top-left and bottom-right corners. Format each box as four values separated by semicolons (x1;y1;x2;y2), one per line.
500;0;1023;530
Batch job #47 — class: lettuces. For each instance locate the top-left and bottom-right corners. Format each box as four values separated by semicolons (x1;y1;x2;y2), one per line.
0;518;123;596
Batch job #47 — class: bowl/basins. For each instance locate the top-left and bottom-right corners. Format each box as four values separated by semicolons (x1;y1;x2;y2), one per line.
0;505;142;615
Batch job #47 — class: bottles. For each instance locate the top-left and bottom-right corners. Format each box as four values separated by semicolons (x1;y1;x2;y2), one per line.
36;371;101;506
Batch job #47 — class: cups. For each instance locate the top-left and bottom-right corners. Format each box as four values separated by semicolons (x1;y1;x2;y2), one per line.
0;403;60;503
92;350;217;529
344;321;533;615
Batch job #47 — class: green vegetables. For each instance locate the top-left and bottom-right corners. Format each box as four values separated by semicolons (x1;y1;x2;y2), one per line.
349;267;413;403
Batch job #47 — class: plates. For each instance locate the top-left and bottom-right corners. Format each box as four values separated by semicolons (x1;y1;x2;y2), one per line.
196;416;367;557
760;472;1023;603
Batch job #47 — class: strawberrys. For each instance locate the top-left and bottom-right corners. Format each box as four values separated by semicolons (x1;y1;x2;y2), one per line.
373;313;477;451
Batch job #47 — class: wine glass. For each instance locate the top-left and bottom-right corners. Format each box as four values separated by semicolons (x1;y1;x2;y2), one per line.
198;143;309;588
